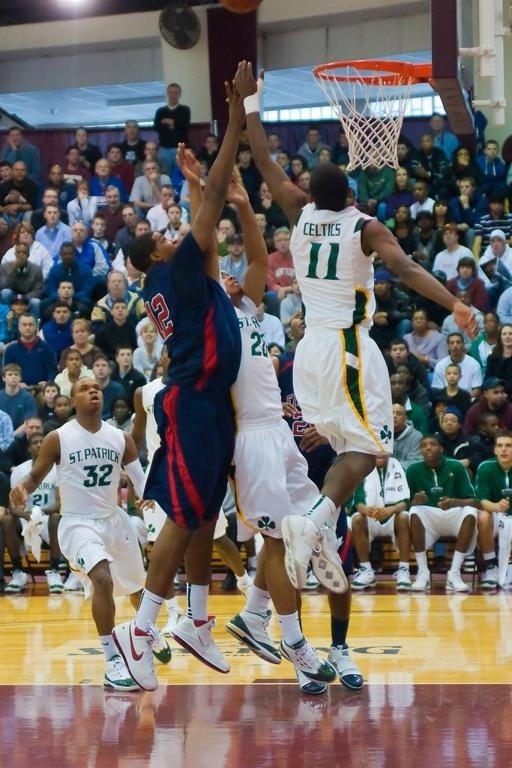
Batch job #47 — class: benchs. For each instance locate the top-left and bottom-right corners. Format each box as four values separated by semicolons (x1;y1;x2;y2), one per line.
379;537;512;579
1;551;73;577
146;539;249;575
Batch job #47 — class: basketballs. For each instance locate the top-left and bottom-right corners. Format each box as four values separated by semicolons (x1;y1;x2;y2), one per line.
221;0;261;14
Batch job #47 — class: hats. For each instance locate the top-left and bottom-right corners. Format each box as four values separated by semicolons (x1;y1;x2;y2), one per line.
12;293;29;305
445;406;464;423
375;271;396;287
481;377;505;390
491;230;506;240
417;211;432;220
226;234;244;244
433;270;447;284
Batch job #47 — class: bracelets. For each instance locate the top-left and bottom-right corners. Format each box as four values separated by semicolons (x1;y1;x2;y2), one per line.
243;95;260;121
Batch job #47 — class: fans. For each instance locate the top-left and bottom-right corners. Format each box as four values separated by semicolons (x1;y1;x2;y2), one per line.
159;0;200;50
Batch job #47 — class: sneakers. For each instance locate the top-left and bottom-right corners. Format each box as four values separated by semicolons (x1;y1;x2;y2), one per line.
479;564;498;589
445;571;469;592
4;570;27;592
310;525;348;594
280;636;337;682
282;516;322;589
152;628;171;663
161;607;184;637
327;643;363;690
45;570;64;592
104;655;139;691
112;620;159;691
294;664;327;695
237;580;254;599
392;567;412;591
225;610;281;664
64;573;79;591
351;566;377;590
411;571;432;591
171;616;229;673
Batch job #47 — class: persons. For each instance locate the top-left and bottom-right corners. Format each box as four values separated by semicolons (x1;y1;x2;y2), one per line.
269;309;368;696
265;125;412;253
337;270;429;589
219;141;302;343
107;79;247;692
104;401;136;432
0;363;75;594
230;57;485;596
132;315;167;383
6;375;172;694
170;140;341;685
402;112;512;595
129;345;253;637
145;83;218;243
1;119;145;400
